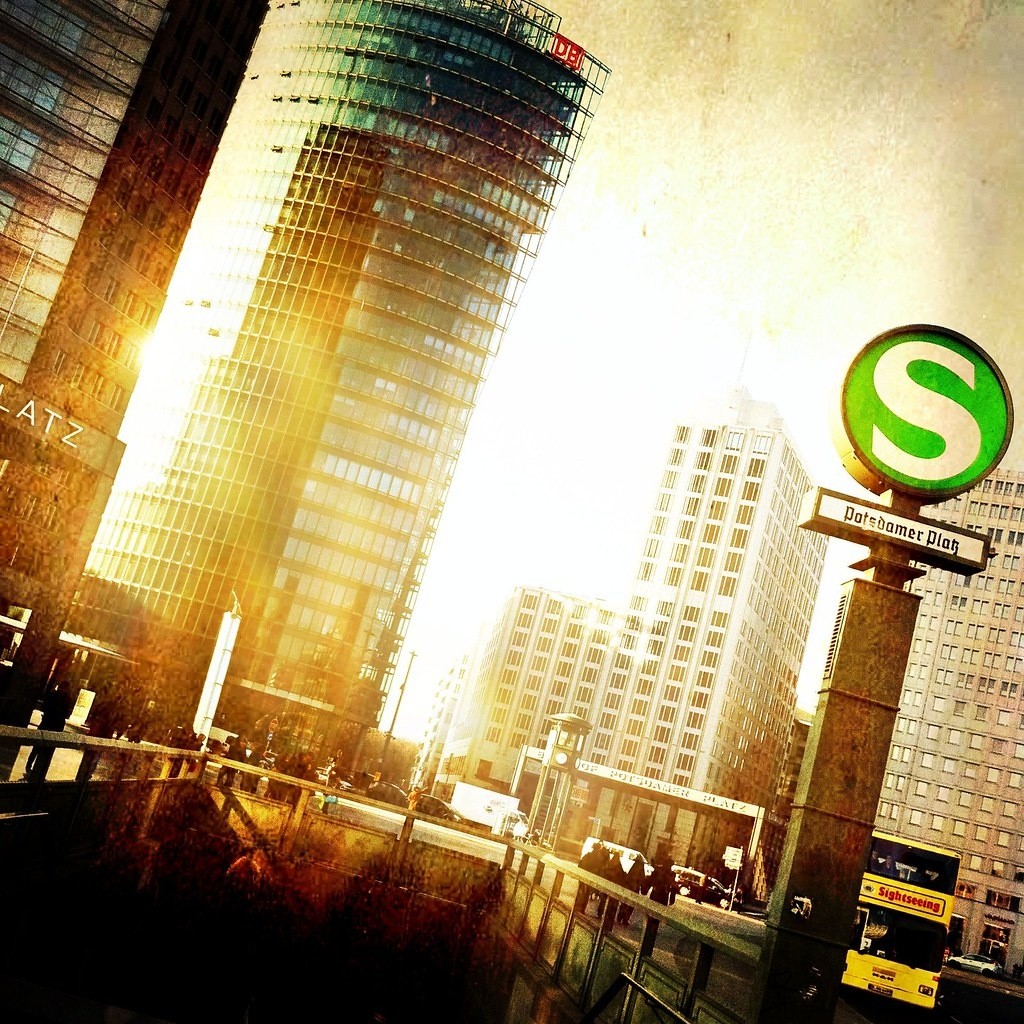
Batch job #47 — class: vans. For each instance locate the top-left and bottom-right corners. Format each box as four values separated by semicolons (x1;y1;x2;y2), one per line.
581;837;654;877
671;864;730;909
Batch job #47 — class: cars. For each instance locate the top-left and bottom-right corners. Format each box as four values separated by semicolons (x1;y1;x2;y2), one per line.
366;780;411;809
948;952;1003;978
313;766;353;791
411;795;465;823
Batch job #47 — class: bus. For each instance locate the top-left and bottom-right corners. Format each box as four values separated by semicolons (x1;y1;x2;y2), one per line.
840;830;963;1009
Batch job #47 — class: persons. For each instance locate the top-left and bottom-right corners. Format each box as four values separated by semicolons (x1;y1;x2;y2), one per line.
867;850;897;876
578;843;677;925
695;874;709;905
408;787;421;810
25;662;205;780
529;832;539;846
910;868;929;884
216;730;342;814
725;884;745;913
513;817;526;841
